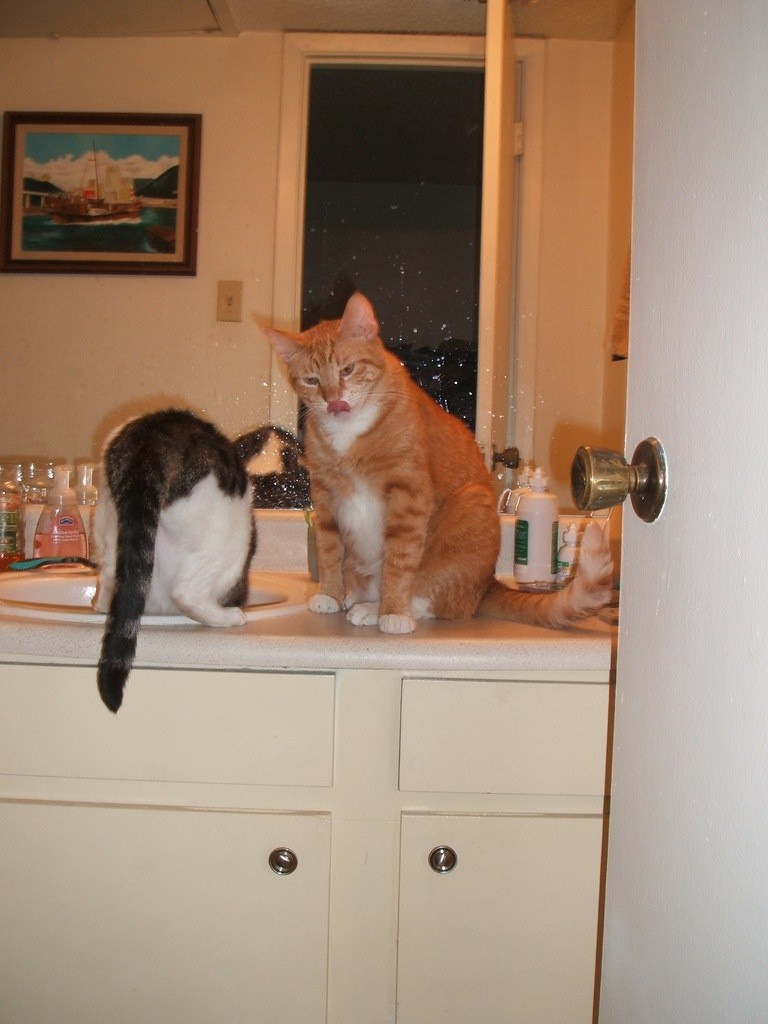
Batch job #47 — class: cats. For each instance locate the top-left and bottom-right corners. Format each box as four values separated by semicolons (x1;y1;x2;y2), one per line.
230;421;309;508
90;408;256;716
256;292;613;636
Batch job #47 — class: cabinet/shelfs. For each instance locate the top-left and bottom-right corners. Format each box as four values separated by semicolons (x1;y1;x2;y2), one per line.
0;654;616;1024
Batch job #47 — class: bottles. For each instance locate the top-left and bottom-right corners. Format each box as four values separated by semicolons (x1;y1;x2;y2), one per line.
0;462;24;571
28;462;54;505
505;466;533;514
512;466;580;586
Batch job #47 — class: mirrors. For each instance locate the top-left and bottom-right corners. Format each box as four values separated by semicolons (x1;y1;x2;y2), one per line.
0;0;635;515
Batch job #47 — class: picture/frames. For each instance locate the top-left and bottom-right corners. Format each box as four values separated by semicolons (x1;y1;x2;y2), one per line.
0;111;202;276
